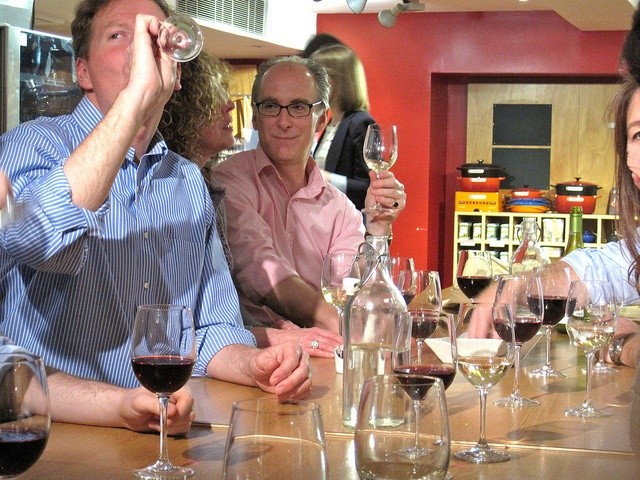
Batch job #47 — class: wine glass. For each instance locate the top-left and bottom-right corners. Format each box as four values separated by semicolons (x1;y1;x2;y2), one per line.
360;120;400;219
526;266;573;380
220;395;331;480
452;303;516;465
0;351;52;480
456;250;493;304
581;282;621;377
157;12;205;64
352;371;451;480
496;274;538;411
323;248;387;337
129;302;198;480
395;268;443;365
384;254;418;308
391;311;457;461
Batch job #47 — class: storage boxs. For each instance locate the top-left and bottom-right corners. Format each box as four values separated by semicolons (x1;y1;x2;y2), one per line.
454;190;506;212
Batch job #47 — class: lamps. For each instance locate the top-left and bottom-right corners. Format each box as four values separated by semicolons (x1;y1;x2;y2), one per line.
377;2;404;28
346;0;367;15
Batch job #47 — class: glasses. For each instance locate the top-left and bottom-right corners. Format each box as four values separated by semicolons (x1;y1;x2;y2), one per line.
251;100;323;118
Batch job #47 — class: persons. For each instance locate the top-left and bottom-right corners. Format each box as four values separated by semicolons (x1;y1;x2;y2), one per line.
214;54;408;336
158;50;235;170
156;48;349;359
467;81;640;366
308;43;380;212
1;163;197;438
0;1;312;401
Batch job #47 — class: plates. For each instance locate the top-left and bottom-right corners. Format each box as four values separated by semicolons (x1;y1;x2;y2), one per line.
504;198;554;213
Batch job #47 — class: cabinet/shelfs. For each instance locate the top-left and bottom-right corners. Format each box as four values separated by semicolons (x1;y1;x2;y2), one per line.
453;211;636;292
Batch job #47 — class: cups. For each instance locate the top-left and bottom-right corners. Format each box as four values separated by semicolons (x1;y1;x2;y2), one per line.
564;278;619;420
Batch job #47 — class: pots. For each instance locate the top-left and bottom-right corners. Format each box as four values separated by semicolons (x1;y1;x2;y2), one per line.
455;159;507;177
455;175;507;193
549;176;604;194
510;183;547;198
555;194;602;214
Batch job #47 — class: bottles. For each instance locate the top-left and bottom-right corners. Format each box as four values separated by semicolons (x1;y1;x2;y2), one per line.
555;204;591;335
341;236;410;431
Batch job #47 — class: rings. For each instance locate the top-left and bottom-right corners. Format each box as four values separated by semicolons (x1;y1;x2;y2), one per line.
390;199;400;211
311;340;320;349
